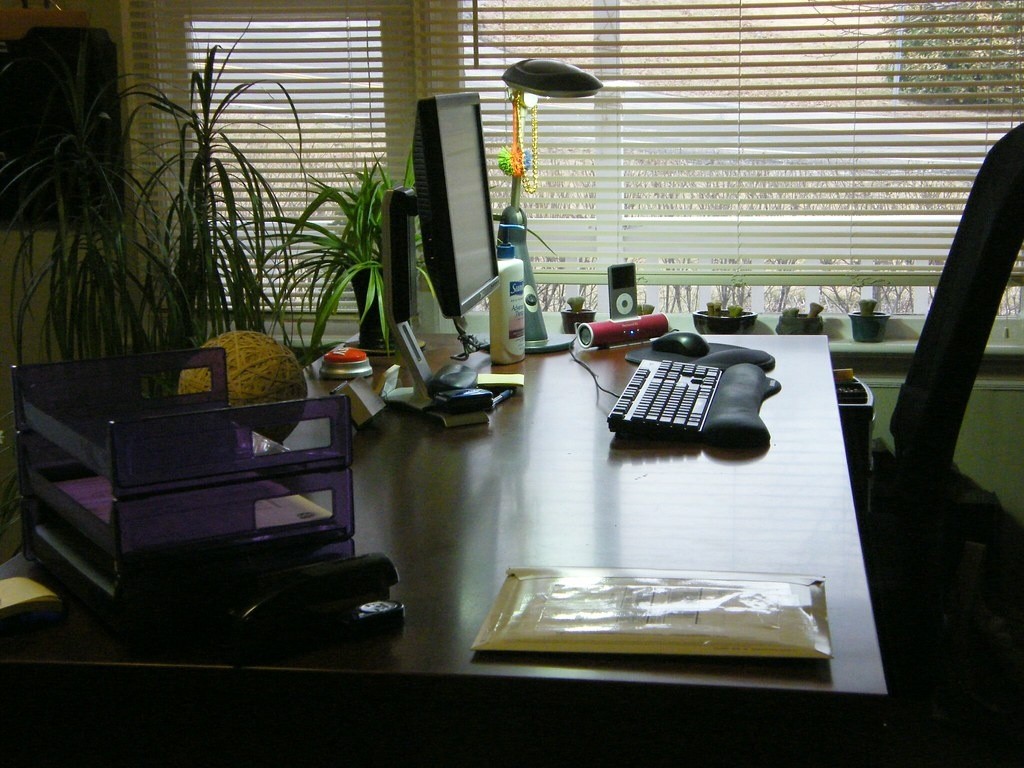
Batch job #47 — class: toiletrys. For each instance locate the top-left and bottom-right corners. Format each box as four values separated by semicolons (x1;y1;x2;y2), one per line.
489;224;526;367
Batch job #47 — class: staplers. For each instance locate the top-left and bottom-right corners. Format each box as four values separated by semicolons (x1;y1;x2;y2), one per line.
213;553;406;672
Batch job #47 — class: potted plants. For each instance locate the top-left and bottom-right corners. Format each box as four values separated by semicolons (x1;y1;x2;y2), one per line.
774;302;824;335
847;300;890;343
560;296;597;333
692;302;758;335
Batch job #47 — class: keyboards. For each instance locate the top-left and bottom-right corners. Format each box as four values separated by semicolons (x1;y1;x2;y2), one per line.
606;360;723;440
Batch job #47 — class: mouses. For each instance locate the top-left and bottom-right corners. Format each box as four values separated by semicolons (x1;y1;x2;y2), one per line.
651;332;710;358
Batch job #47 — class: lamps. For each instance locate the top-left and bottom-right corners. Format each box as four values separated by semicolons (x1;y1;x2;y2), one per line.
498;59;604;353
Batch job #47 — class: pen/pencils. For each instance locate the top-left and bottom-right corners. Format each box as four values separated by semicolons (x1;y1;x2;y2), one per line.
492;390;514;407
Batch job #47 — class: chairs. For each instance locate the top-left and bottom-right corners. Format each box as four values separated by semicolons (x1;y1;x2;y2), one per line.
848;124;1024;768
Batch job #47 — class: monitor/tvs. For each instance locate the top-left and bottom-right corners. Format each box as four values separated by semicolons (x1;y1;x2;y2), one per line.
382;93;501;429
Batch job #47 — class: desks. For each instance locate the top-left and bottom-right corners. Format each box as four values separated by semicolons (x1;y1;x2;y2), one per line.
0;332;888;721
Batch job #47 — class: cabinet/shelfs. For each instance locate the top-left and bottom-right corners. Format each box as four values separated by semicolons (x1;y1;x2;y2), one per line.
11;346;356;643
